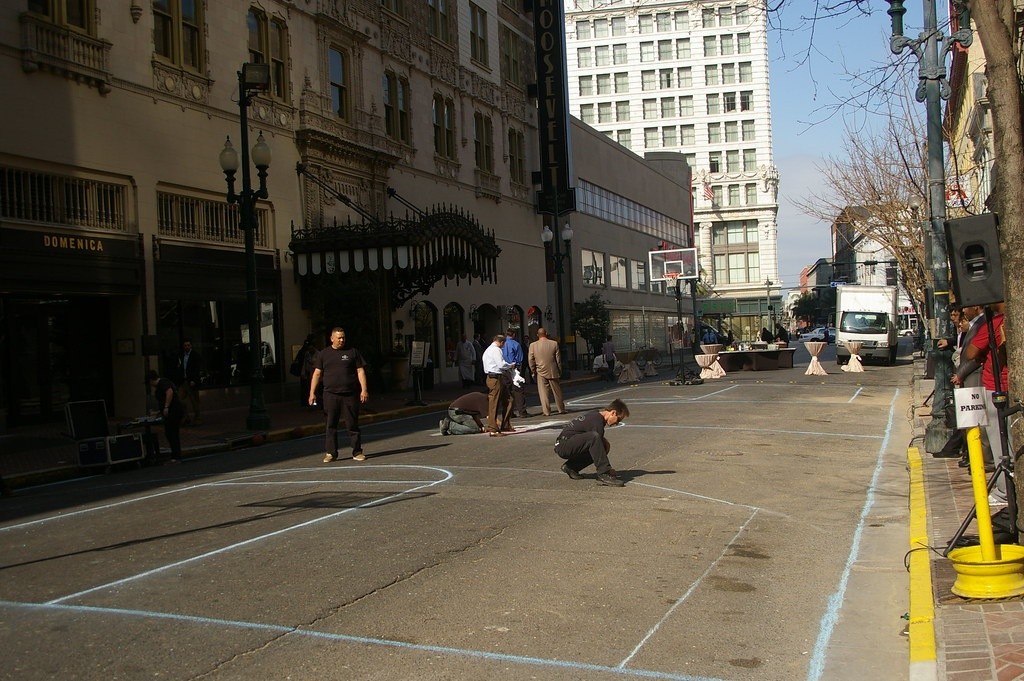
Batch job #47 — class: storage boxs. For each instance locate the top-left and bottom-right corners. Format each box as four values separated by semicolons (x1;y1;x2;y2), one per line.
64;399;144;468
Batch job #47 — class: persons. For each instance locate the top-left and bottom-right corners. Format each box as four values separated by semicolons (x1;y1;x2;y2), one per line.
439;392;512;436
933;302;1014;519
473;333;493;385
824;327;829;346
774;323;788;348
453;333;476;389
501;328;534;418
761;328;773;344
702;327;718;345
308;327;369;463
717;329;726;351
602;335;617;382
528;328;568;416
554;397;630;487
144;369;184;461
728;330;733;345
176;338;201;425
690;329;695;359
297;333;320;408
522;335;537;384
482;334;515;437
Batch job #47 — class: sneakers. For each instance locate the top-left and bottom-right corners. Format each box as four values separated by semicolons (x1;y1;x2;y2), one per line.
323;451;338;463
353;453;366;461
596;473;624;487
561;462;584;479
439;418;449;436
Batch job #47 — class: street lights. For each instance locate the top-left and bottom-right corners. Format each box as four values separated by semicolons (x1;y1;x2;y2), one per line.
542;184;573;380
218;61;273;414
909;180;934;379
685;238;702;359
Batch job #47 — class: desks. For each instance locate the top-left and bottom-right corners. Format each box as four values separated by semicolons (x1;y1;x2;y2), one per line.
614;349;659;383
717;343;797;372
841;342;865;372
804;342;828;375
694;344;726;379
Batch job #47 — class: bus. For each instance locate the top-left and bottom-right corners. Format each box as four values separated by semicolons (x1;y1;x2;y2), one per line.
897;312;920;336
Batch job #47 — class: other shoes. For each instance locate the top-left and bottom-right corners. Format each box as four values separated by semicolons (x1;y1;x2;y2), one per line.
510;413;516;418
490;431;505;436
932;448;994;475
501;426;516;432
520;413;533;418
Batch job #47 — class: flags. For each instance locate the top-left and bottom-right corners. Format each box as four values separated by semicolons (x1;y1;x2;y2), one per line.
704;183;714;201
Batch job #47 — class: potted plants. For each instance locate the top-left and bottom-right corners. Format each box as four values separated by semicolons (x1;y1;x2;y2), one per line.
388;319;409;391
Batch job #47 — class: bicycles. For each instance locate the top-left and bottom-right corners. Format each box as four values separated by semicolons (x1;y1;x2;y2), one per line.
633;346;664;367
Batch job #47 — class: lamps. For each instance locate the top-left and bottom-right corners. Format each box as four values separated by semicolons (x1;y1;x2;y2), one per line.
469;303;480;322
544;304;553;321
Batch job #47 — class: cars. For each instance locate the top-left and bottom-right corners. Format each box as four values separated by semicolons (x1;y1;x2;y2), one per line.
800;327;836;342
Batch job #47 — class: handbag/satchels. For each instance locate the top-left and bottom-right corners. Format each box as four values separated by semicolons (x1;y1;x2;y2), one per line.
489;372;498;378
166;382;188;423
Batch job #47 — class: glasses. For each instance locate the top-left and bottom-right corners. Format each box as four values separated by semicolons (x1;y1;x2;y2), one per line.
616;414;622;422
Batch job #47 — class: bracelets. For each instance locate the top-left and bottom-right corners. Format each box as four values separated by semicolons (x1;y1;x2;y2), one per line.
165;406;169;408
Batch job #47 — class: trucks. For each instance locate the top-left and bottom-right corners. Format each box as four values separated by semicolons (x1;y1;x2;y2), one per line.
836;284;899;366
